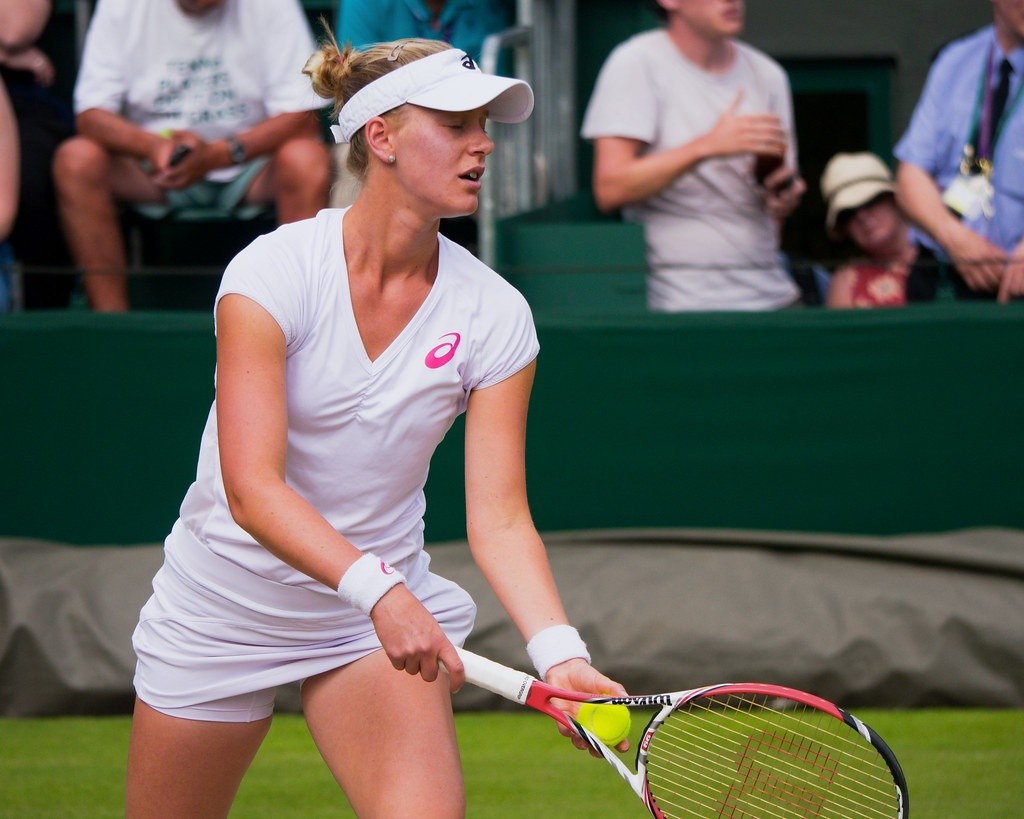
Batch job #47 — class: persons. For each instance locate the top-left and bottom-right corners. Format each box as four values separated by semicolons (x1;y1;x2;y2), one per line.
0;0;516;315
580;1;804;317
805;154;970;308
123;33;630;819
892;0;1024;303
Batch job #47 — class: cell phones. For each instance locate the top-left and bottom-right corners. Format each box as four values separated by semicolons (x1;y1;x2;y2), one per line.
168;144;191;167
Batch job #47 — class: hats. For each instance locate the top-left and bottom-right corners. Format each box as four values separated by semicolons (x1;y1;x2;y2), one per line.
817;150;898;238
328;45;536;148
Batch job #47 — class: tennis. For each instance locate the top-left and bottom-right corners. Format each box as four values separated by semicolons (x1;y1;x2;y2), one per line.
575;694;631;749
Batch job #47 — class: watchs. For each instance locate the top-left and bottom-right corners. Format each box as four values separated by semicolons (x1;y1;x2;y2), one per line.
226;135;246;164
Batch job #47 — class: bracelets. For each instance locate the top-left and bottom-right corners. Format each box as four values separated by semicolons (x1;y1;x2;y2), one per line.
526;624;592;682
337;552;407;617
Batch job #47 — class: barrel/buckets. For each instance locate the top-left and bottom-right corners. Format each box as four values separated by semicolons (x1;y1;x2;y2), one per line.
497;218;648;314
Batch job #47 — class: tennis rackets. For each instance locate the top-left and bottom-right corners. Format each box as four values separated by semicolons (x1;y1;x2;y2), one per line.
438;645;911;819
